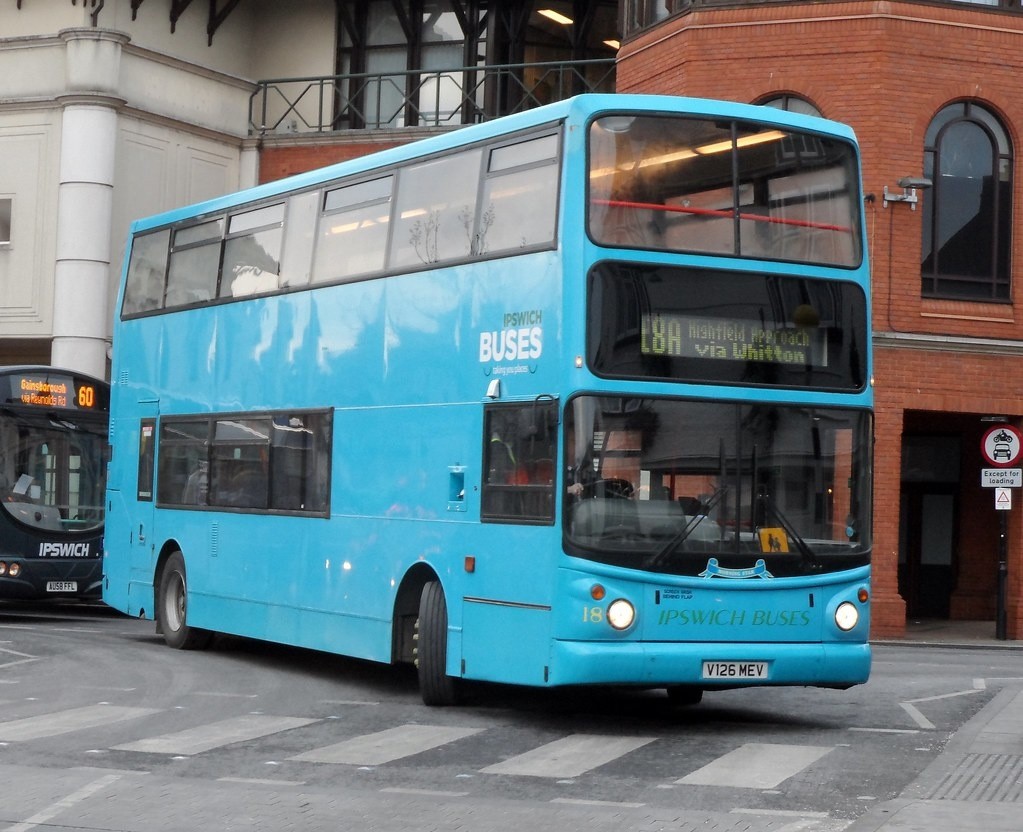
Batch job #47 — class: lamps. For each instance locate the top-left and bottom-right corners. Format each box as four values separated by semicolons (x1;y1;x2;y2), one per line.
883;177;932;210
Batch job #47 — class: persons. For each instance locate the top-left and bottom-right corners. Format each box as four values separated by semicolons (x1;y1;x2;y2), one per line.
182;447;289;507
506;428;585;496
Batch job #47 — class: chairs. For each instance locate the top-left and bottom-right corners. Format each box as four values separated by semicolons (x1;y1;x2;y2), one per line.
161;458;561;518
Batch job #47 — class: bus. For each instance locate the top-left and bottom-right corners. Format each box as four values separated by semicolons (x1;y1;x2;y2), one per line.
99;91;876;708
0;364;113;607
